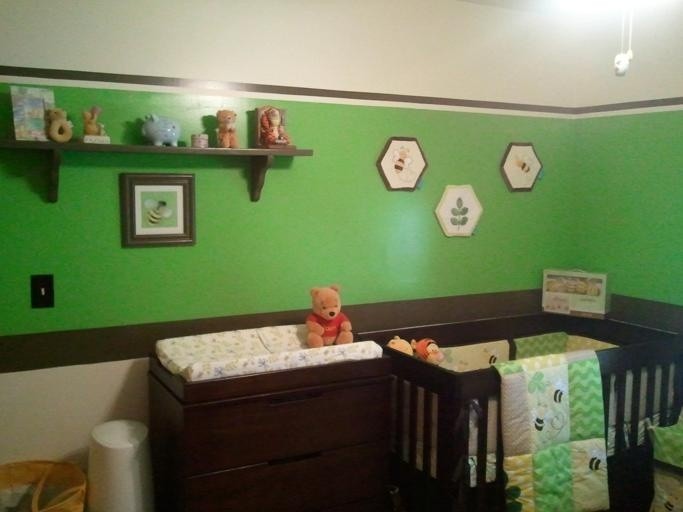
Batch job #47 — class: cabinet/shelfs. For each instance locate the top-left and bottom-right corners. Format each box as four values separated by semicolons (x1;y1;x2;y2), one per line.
147;351;402;512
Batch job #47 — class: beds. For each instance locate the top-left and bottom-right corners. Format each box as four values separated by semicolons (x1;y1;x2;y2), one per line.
355;311;682;512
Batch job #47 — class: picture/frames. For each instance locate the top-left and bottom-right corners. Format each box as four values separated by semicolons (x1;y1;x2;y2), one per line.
499;142;542;193
118;172;196;248
9;85;56;141
433;184;483;237
375;136;427;192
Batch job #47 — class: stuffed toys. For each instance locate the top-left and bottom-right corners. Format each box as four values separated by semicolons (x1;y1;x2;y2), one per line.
388;335;413;356
216;109;238;148
411;338;445;366
48;108;72;141
83;106;111;143
305;284;353;349
